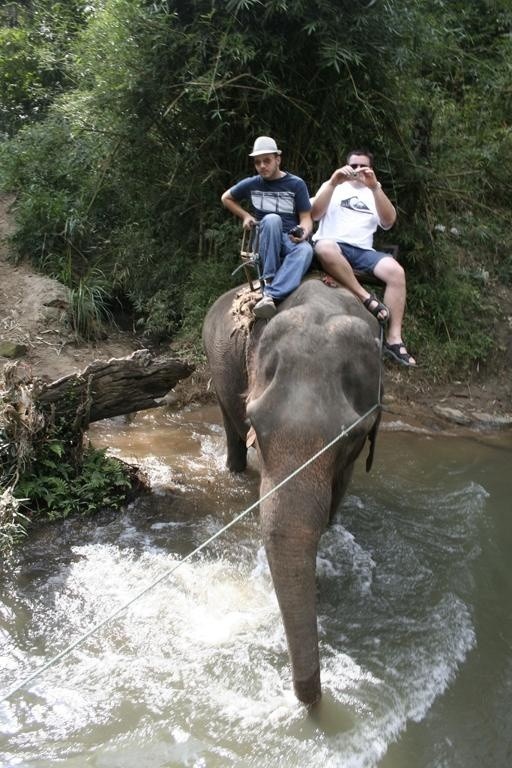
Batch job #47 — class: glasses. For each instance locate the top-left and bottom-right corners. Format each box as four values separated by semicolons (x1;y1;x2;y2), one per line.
350;164;369;170
254;157;276;166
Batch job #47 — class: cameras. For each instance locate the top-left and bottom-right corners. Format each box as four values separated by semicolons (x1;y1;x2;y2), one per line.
347;170;360;180
288;226;304;238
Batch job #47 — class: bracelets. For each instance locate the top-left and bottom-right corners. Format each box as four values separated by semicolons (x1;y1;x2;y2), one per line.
367;180;382;193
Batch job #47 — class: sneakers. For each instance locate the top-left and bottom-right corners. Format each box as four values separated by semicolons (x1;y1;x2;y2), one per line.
253;296;276;319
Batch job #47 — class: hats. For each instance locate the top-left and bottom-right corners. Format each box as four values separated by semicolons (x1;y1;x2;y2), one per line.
248;136;283;156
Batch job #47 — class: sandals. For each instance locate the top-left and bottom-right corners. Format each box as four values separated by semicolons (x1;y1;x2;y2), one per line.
364;292;389;323
383;335;417;367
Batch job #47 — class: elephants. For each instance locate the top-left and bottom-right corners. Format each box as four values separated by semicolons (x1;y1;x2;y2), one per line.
202;271;384;705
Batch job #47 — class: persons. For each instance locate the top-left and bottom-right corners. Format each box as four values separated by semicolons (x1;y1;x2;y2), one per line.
309;149;418;367
220;135;315;321
